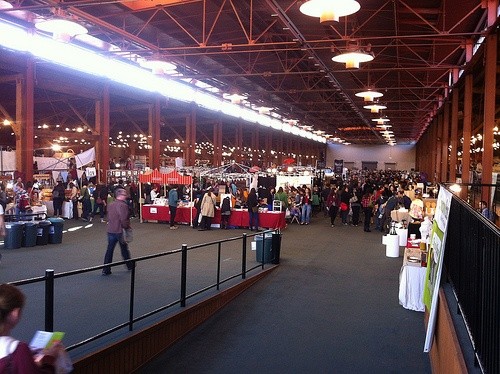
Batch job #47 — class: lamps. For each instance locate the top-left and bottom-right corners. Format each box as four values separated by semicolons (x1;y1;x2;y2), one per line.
0;0;398;146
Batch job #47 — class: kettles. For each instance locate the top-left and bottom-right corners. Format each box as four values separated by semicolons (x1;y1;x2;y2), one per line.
390;226;397;235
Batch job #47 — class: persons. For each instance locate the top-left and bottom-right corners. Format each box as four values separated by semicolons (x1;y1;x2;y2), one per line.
0;283;62;374
0;166;438;236
100;189;136;276
479;201;488;218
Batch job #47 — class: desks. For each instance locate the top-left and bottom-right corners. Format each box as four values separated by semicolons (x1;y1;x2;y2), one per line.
141;203;287;234
398;236;428;313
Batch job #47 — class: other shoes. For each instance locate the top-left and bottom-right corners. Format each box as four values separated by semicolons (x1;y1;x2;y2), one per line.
167;220;262;232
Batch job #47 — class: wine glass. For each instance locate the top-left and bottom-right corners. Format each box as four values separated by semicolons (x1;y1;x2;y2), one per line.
410;234;416;243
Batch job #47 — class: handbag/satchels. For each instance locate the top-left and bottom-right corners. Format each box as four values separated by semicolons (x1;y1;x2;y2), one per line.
96;198;104;206
79;195;85;204
339;201;348;213
52;191;59;200
251;207;258;214
349;196;358;203
71;195;77;203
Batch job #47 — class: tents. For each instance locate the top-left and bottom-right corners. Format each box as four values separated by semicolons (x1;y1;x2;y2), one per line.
199;160;263;190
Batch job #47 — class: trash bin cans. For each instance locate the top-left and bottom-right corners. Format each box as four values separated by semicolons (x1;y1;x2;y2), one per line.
19;221;39;248
36;220;52;246
269;232;283;259
47;217;65;244
4;222;25;249
254;234;273;263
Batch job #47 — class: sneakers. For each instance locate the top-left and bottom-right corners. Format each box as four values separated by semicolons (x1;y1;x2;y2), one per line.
100;269;113;276
128;263;137;272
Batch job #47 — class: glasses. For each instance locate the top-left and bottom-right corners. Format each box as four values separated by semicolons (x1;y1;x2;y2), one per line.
117;192;127;197
